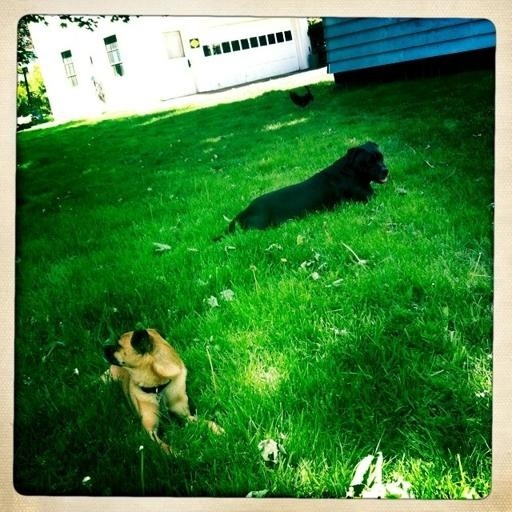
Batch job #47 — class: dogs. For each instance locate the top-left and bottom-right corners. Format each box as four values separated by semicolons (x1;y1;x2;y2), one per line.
85;328;227;456
213;141;389;243
